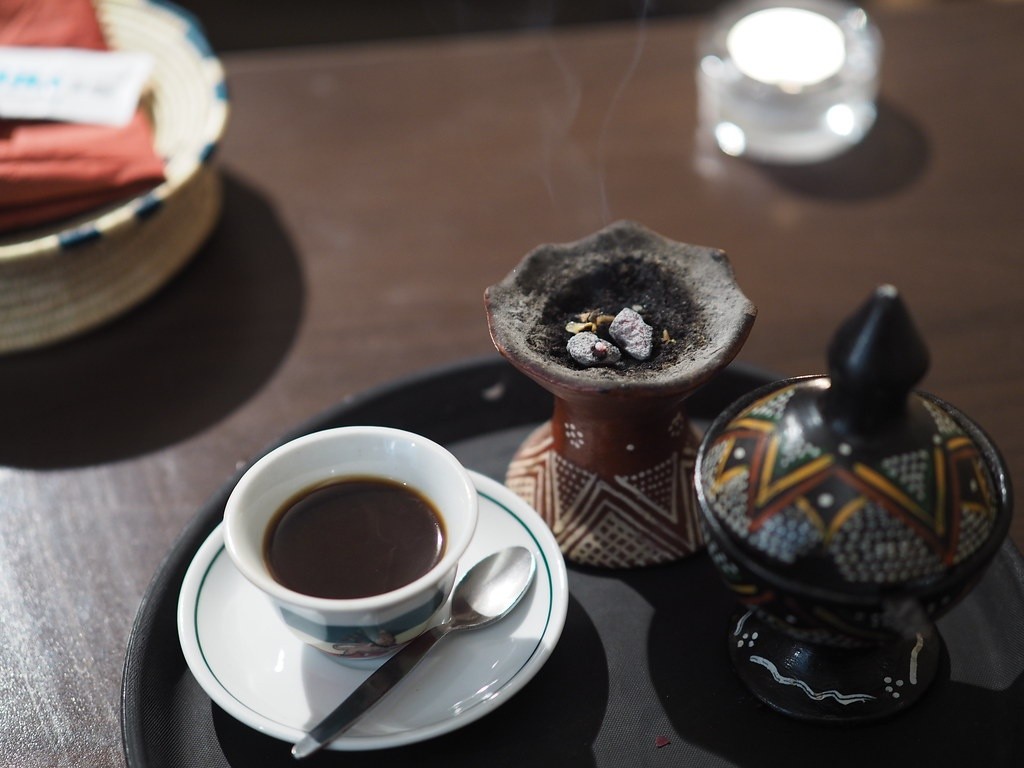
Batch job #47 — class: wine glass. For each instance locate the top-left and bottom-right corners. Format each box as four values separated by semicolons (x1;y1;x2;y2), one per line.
696;286;1009;733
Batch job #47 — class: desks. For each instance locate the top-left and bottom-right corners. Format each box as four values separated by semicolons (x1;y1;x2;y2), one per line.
0;1;1024;768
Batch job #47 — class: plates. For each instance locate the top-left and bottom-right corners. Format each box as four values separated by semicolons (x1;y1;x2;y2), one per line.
2;170;220;349
177;468;568;751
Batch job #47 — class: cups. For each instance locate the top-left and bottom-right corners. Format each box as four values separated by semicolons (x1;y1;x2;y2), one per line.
224;426;479;660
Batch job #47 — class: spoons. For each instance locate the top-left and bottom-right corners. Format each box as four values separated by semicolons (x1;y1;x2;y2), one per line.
291;545;537;759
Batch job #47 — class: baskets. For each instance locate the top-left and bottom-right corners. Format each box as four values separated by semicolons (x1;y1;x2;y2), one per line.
0;0;230;354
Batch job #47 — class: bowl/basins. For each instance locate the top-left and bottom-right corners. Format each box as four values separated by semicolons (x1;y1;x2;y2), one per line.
696;1;881;162
0;0;231;272
